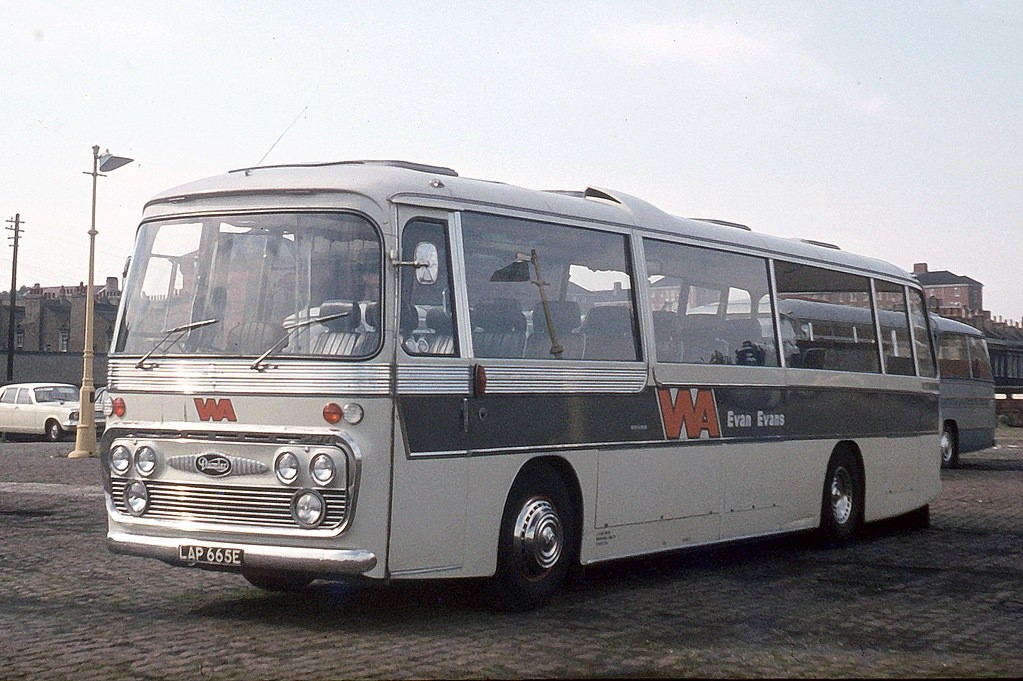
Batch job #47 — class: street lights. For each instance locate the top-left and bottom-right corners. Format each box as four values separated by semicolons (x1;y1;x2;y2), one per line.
67;145;135;460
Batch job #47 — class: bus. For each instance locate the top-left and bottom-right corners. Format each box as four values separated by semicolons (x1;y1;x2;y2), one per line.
99;159;944;616
914;338;1023;427
682;297;998;472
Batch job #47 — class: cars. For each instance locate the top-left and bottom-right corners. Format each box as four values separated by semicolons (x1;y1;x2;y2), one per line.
94;387;107;435
0;382;106;443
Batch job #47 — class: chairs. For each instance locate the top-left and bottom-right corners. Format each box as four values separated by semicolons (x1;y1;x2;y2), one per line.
228;297;827;368
